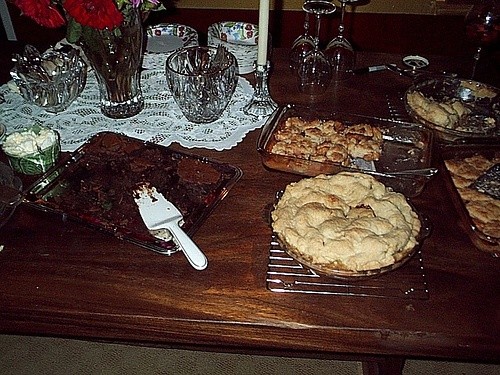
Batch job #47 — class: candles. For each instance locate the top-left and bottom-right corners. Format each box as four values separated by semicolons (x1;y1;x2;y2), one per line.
258;0;269;65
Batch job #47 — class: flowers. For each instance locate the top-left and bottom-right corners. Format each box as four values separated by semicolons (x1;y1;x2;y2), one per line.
13;0;166;44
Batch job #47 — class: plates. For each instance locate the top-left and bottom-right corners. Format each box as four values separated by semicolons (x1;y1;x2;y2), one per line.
142;23;199;69
403;78;500;140
207;21;272;74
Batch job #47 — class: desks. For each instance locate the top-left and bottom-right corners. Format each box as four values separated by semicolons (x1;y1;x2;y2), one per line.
0;7;500;374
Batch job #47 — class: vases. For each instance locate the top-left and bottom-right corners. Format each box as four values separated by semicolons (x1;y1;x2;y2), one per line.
76;11;145;120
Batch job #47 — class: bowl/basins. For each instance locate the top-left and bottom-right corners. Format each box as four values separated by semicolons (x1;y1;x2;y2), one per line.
10;46;87;113
434;141;500;256
1;126;61;176
414;71;461;103
256;103;434;199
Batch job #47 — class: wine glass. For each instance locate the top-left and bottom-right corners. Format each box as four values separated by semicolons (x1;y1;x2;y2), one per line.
296;1;337;94
462;0;500;65
324;0;360;82
289;0;318;75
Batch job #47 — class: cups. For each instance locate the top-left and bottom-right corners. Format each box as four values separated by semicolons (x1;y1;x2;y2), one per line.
165;46;239;123
